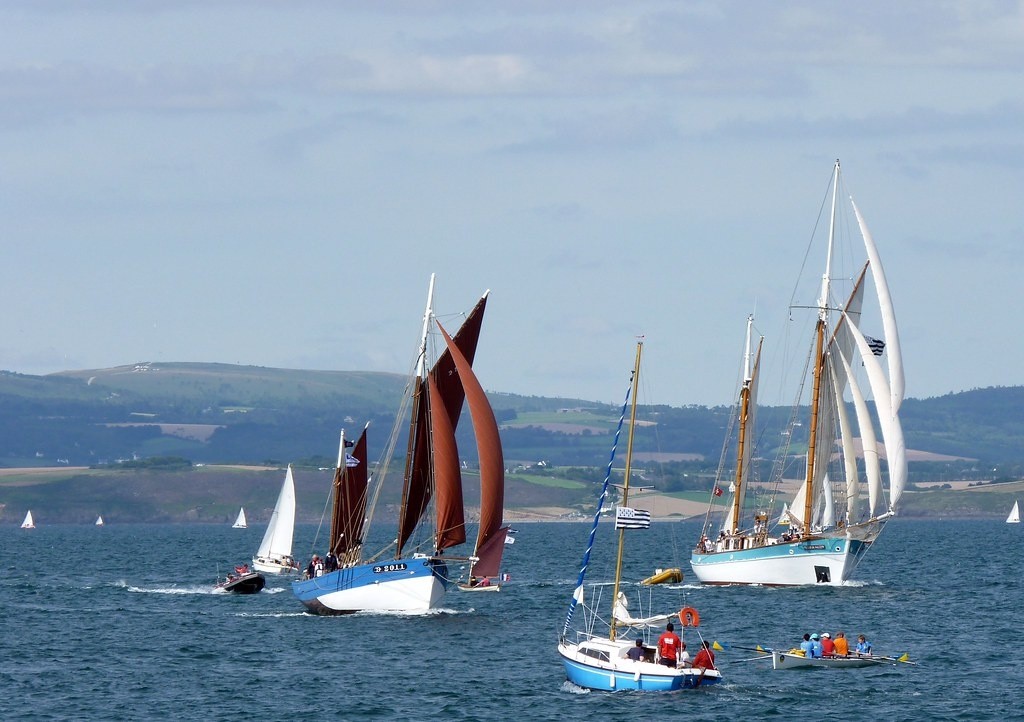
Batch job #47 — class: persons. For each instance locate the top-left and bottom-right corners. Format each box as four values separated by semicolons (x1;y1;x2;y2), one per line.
856;634;873;657
470;576;479;587
832;632;849;659
657;623;681;669
701;520;800;553
473;575;490;588
800;633;814;658
625;639;645;662
820;633;835;657
809;633;824;658
692;640;715;670
222;548;339;587
675;643;689;668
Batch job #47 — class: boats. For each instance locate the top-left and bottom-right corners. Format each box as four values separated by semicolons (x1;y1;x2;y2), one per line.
223;572;266;594
641;568;683;585
771;652;882;671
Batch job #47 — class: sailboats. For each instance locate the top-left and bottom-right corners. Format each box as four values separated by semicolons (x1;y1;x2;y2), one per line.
688;156;911;589
95;516;104;528
231;507;249;529
553;334;725;694
20;510;36;529
251;462;299;575
1005;500;1021;524
290;271;520;617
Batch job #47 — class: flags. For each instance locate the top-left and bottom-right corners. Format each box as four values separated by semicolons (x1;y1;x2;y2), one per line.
862;334;885;356
498;573;511;582
714;486;724;498
345;453;360;468
614;506;650;532
343;439;354;448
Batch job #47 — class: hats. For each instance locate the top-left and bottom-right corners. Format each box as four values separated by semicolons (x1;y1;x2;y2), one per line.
821;633;830;638
810;633;819;640
781;533;787;536
312;555;318;558
836;631;844;636
804;634;810;638
636;639;643;643
